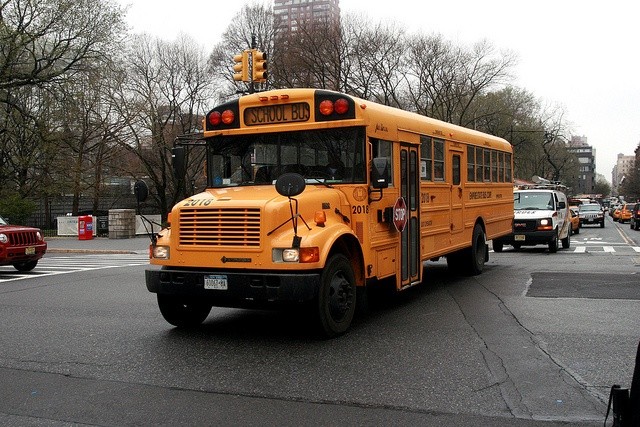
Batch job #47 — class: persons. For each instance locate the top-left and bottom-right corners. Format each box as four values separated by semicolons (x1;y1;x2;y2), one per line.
322;148;346;179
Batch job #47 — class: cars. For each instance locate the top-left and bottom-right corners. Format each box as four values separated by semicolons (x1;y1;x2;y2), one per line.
602;197;623;221
568;206;580;235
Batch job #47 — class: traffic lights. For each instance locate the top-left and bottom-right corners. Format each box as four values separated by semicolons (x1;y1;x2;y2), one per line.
233;51;248;82
252;49;267;81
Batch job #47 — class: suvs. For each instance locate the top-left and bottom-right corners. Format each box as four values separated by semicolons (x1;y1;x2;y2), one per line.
493;183;570;253
0;213;47;272
618;203;637;223
578;203;604;228
630;204;640;230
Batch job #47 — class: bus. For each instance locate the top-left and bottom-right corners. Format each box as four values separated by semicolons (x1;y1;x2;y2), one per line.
568;194;603;205
145;88;515;336
